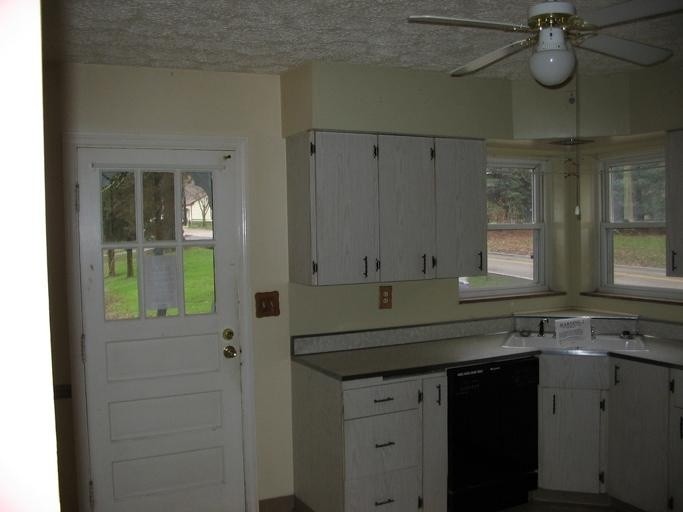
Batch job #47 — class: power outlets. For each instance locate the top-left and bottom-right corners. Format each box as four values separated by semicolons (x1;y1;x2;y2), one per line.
378;285;392;310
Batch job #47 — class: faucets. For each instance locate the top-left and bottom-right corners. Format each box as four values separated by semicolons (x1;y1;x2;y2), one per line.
537;318;549;336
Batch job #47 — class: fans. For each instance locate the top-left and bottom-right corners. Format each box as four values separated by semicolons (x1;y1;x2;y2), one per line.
408;0;683;78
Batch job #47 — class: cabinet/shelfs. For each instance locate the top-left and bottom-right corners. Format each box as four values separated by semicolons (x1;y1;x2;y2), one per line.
535;353;608;495
286;129;488;287
288;359;448;512
605;355;683;512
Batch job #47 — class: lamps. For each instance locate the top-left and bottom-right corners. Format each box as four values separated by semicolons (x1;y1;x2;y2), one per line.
528;28;576;86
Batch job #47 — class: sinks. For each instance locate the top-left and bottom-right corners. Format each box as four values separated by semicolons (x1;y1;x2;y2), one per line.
502;331;650;352
